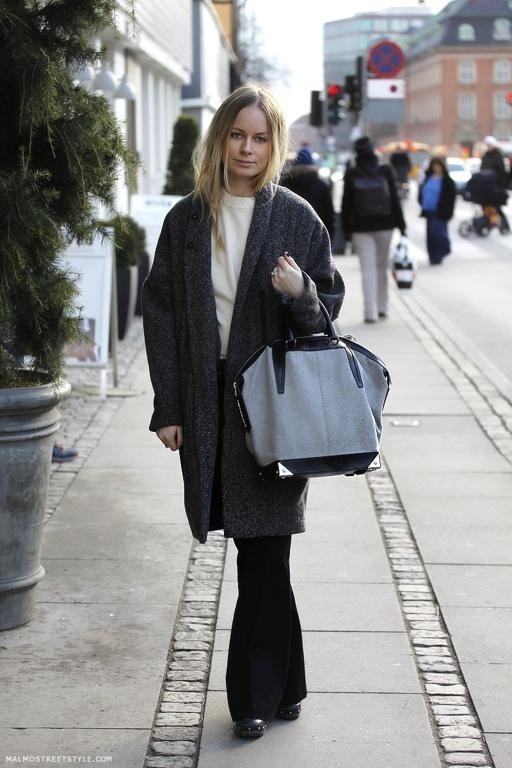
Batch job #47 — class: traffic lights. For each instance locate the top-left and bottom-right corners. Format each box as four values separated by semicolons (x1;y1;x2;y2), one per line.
347;73;362;111
326;81;345;125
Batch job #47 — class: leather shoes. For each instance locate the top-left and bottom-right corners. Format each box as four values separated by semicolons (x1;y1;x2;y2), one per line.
236;700;302;736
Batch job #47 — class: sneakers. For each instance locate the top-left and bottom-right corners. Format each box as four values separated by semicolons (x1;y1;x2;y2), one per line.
52;446;78;462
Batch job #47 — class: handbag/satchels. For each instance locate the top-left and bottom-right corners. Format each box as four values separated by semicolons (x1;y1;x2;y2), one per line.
392;235;417;288
354;176;392;215
233;297;390;480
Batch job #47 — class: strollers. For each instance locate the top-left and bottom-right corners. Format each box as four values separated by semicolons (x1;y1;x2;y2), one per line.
455;167;512;240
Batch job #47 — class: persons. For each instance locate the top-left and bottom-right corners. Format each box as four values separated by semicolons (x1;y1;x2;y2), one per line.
418;154;456;265
479;136;511;234
390;146;412;184
340;138;406;324
140;85;344;738
278;146;336;247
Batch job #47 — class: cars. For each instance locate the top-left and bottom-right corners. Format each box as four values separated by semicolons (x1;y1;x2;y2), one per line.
277;138;512;196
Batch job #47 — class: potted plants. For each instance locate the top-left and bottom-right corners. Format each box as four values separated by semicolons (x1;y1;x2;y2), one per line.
0;0;144;629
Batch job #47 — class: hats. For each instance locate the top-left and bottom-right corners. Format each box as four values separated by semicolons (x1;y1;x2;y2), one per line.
294;150;313;165
354;137;373;155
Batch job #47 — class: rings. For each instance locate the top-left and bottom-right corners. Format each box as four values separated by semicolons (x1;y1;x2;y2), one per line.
271;271;277;278
